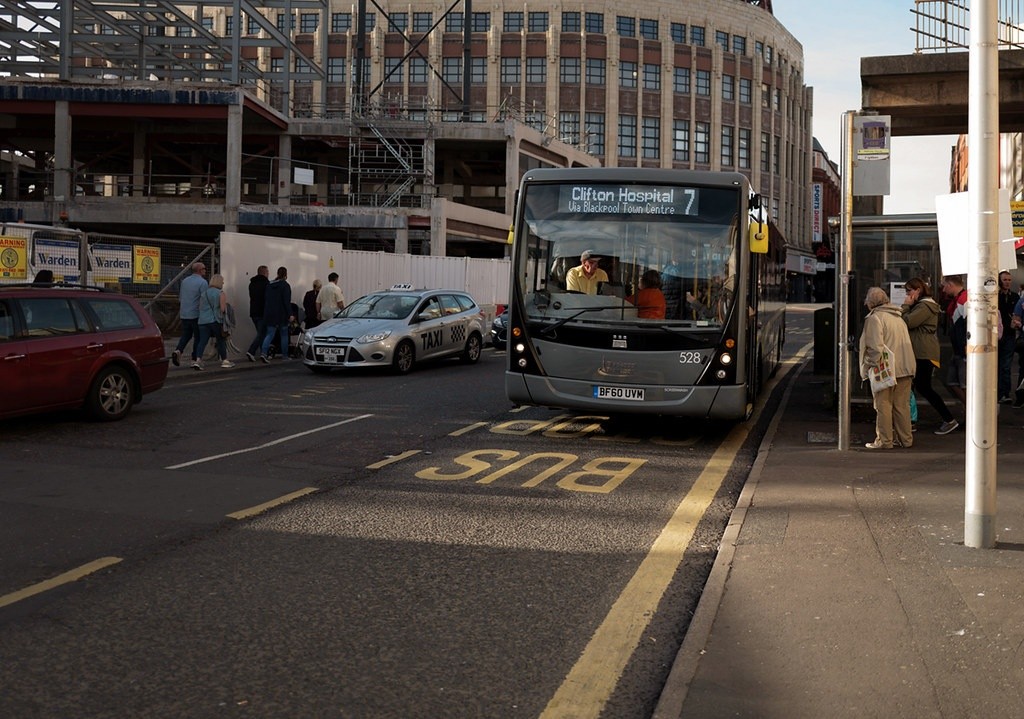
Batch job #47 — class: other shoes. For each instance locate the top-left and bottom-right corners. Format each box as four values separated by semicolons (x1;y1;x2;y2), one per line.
865;442;894;449
998;395;1013;404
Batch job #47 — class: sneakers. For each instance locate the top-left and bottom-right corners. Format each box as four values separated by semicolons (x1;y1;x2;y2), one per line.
221;361;235;367
246;352;256;362
934;419;959;434
912;423;917;432
191;361;205;371
260;355;270;363
280;355;292;361
171;350;180;367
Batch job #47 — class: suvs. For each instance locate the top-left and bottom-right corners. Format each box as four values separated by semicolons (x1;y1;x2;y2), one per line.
1;280;169;420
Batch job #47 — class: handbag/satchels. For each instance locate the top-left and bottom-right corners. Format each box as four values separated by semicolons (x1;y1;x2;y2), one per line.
868;343;897;393
220;326;231;337
910;390;918;421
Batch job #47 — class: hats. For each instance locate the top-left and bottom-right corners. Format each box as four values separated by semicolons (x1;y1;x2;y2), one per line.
581;250;603;261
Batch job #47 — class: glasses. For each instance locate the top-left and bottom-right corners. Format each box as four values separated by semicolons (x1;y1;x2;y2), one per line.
202;267;206;270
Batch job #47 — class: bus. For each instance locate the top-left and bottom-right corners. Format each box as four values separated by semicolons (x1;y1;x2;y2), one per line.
508;164;791;424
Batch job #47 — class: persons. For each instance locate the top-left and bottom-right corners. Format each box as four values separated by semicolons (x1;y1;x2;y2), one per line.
170;264;235;371
857;271;1024;451
663;251;726;326
245;266;345;364
565;249;609;296
32;270;55;287
625;270;667;319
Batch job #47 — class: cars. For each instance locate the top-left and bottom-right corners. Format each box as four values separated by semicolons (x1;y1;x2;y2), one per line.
301;284;487;374
490;303;509;349
